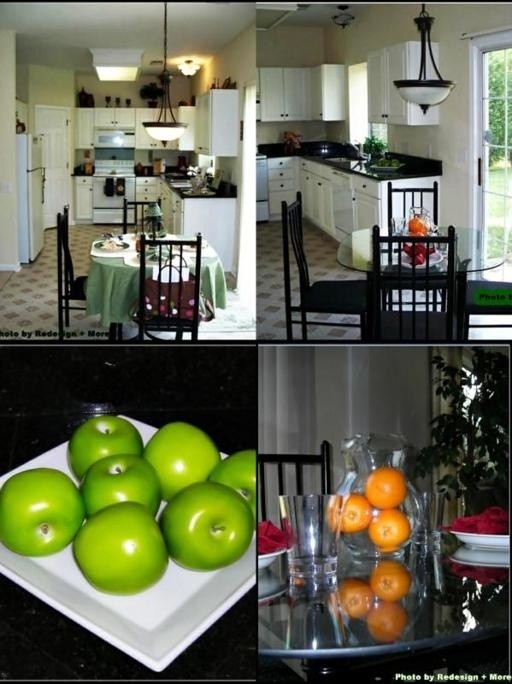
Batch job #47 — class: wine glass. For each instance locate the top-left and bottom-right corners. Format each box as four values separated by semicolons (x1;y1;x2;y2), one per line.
105;95;132;106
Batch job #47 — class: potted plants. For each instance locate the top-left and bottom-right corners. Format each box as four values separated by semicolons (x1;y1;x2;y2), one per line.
364;136;387;160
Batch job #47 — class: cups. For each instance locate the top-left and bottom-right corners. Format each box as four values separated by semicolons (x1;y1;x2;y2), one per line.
283;572;347;650
275;491;343;578
392;217;407;235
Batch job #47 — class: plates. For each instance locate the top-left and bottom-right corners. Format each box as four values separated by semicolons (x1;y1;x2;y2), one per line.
446;529;509;550
449;545;512;569
395;249;443;271
91;240;209;268
369;163;407;172
1;410;256;668
256;574;289;600
257;550;286;571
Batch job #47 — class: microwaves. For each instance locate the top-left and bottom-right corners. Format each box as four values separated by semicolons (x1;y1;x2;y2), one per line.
94;129;135;148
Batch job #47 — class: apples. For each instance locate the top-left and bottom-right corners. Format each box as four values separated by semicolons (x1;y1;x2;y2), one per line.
1;467;86;557
408;218;424;233
73;501;169;596
339;578;377;617
340;495;371;531
368;602;408;643
66;416;143;482
365;467;407;508
209;449;256;527
370;560;412;601
79;454;162;518
159;481;255;571
369;510;410;549
140;421;221;501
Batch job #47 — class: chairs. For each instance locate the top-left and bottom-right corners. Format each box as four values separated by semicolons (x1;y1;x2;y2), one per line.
281;181;512;341
257;440;332;560
56;196;203;342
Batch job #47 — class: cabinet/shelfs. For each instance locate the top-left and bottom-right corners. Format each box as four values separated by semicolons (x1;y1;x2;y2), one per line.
268;159;440;243
74;89;240;272
256;41;439;126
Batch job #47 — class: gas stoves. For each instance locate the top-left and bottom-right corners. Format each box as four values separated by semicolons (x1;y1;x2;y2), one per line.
93;171;136;175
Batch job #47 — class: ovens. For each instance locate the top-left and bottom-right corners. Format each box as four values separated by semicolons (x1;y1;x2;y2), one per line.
93;177;137;224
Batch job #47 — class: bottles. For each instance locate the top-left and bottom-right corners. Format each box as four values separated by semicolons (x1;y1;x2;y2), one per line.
210;76;231;88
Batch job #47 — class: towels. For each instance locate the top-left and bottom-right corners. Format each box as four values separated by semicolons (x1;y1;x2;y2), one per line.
258;520;295;555
441;561;508;584
443;508;509;535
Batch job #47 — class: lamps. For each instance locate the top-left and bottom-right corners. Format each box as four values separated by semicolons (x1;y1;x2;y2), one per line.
141;1;190;148
332;6;355;28
392;4;457;116
87;47;146;83
177;59;200;78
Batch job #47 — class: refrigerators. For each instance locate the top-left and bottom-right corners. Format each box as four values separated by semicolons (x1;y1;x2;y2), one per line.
15;133;44;271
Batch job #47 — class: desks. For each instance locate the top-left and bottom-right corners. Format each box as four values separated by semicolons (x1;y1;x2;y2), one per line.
258;551;510;684
